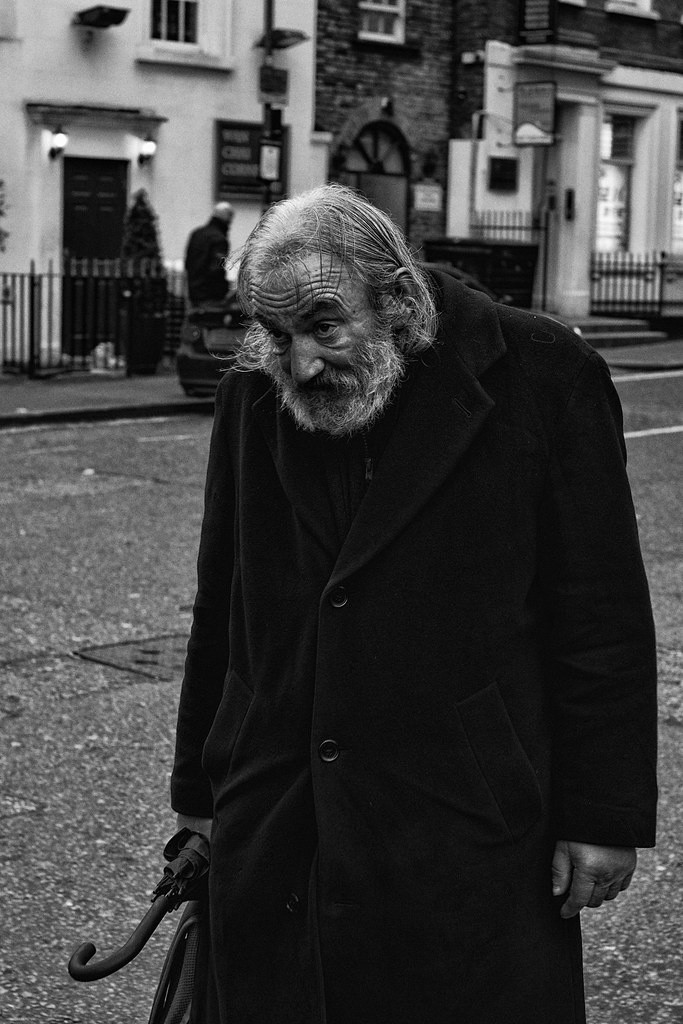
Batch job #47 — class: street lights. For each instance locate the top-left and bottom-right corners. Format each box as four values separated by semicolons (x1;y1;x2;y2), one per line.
256;29;310;214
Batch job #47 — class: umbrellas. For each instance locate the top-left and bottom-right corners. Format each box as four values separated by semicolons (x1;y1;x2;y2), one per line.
66;827;211;982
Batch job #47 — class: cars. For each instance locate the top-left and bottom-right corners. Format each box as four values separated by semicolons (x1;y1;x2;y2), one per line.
176;262;580;397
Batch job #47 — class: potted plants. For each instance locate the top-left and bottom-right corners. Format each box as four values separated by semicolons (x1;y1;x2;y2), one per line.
119;189;169;375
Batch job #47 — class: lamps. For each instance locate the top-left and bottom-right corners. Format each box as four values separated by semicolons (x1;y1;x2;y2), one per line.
255;29;310;48
48;124;68;158
72;6;129;28
139;132;158;165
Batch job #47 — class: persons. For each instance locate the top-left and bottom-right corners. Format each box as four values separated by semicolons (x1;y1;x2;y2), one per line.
168;183;657;1024
185;201;233;303
